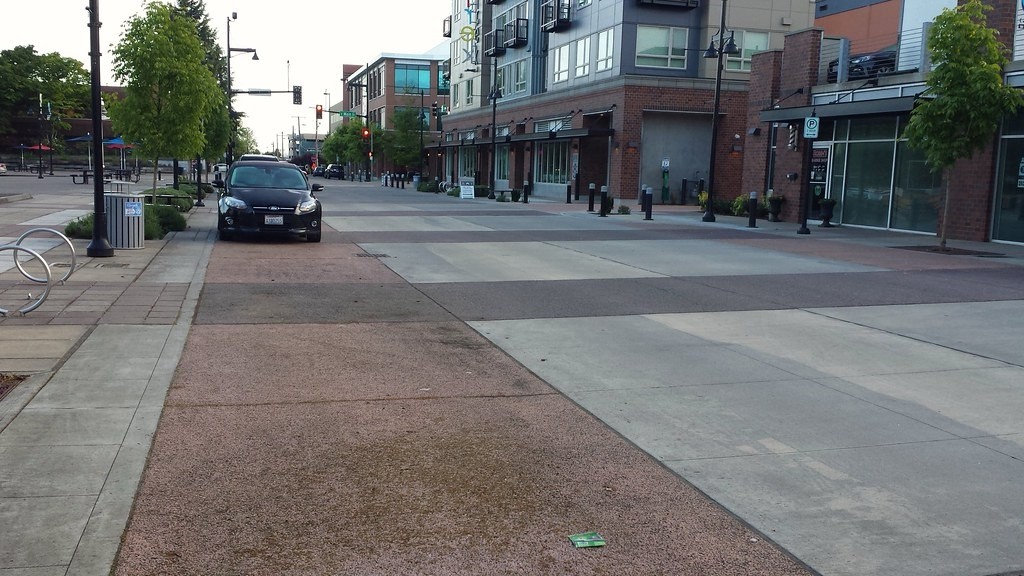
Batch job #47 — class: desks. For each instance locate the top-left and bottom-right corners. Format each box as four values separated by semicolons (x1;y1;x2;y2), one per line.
78;169;96;184
118;169;136;181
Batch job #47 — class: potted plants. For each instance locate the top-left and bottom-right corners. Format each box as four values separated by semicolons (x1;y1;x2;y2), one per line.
761;193;783;221
817;198;836;227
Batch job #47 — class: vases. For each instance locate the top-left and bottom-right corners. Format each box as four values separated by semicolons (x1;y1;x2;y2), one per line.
699;204;706;210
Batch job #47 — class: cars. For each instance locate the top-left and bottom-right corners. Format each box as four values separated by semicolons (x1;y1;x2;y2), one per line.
237;154;279;162
212;164;229;172
211;161;325;242
298;166;310;174
313;166;324;177
0;163;7;174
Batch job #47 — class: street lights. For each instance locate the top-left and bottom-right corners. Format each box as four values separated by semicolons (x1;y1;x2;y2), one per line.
486;83;503;200
324;93;332;134
35;114;46;179
298;124;306;155
48;116;60;174
341;78;370;182
702;27;739;222
417;112;426;183
227;48;259;169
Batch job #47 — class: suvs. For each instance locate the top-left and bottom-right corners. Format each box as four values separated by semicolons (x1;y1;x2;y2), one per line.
323;164;345;181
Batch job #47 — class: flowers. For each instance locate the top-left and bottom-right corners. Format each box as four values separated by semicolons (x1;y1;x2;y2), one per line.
698;191;708;204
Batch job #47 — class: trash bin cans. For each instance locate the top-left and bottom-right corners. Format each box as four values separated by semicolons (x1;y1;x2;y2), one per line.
412;175;420;189
104;178;147;251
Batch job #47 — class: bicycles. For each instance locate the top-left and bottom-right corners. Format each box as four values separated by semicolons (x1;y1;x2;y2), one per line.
439;179;459;194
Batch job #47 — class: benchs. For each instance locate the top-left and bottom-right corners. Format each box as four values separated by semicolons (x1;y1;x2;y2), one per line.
30;165;50;173
488;189;522;201
157;171;177;180
137;193;191;212
6;164;31;172
179;182;211;189
113;174;141;183
70;172;113;185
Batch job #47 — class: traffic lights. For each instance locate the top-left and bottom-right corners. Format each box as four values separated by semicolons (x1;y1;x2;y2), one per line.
369;152;372;156
363;128;369;143
432;101;438;117
316;105;322;119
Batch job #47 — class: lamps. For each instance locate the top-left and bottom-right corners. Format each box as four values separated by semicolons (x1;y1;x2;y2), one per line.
611;141;620;148
571;143;578;149
524;146;531;151
627;141;638;149
748;127;760;135
731;145;742;152
